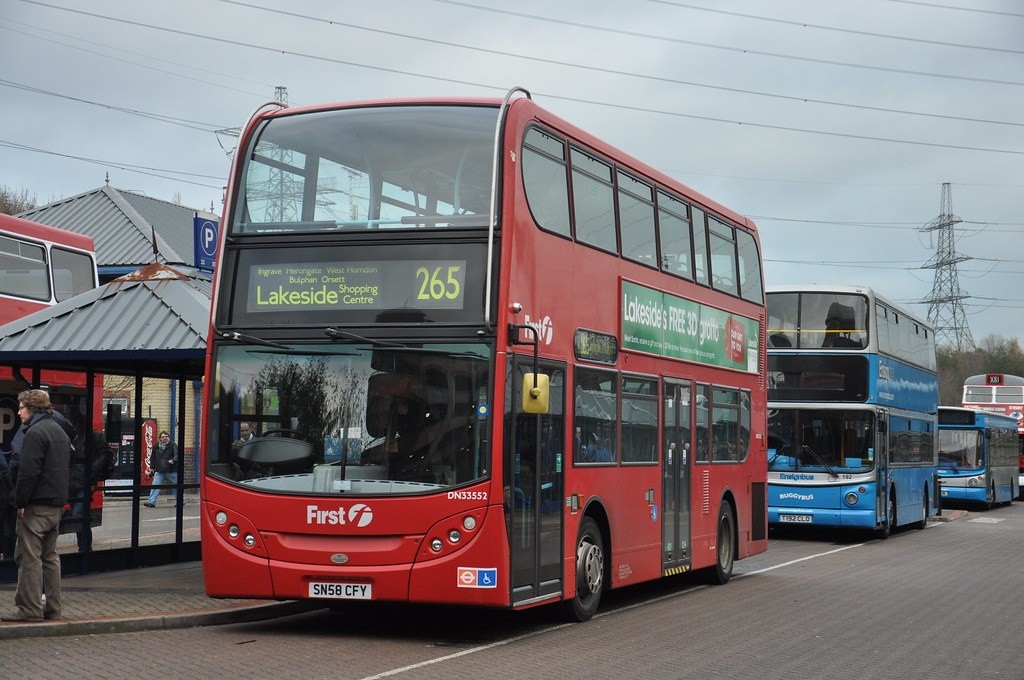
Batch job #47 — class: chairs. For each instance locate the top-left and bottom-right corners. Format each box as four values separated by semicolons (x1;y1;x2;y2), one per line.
672;261;735;294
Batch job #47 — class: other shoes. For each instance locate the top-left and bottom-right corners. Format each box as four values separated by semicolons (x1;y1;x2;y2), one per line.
174;501;186;507
43;611;62;620
0;614;44;622
143;500;155;508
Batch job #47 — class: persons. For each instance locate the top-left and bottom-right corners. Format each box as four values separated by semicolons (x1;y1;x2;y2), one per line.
238;421;257;444
0;388;117;623
143;431;186;508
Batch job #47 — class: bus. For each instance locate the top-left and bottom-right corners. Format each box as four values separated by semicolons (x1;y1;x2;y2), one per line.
767;284;939;541
201;85;768;624
937;405;1020;511
0;212;105;533
962;373;1024;501
